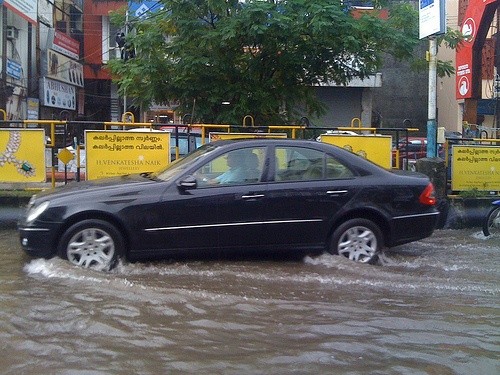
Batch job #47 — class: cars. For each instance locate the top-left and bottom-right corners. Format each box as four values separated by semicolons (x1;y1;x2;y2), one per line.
391;138;449;169
17;137;440;275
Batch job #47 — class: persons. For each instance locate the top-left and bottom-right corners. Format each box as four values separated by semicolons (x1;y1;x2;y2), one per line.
192;150;248;187
116;32;127;59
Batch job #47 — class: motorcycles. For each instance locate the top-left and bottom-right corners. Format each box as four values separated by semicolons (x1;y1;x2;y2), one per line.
482;200;500;236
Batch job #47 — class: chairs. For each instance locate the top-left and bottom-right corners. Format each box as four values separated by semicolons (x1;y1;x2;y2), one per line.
244;153;260;183
